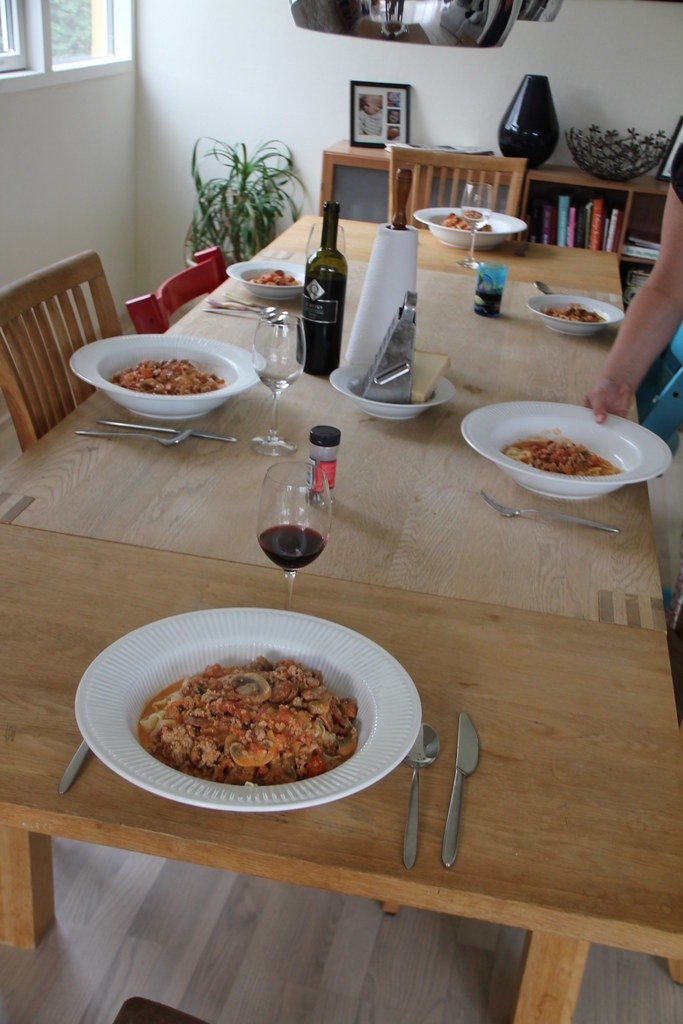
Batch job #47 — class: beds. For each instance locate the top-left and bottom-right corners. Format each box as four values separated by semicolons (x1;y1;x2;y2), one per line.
439;4;483;42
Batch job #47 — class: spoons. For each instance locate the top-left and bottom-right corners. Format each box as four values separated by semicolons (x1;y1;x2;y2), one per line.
75;428;192;446
535;281;554;295
403;723;440;869
203;307;276;315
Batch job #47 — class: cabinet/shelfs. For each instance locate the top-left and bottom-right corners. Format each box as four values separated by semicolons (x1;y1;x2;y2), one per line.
319;140;669;310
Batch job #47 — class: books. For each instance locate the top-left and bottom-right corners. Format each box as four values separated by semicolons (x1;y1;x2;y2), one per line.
622;231;663;261
521;194;625;253
623;270;649;305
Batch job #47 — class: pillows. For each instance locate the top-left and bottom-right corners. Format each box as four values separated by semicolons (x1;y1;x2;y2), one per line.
468;11;484;24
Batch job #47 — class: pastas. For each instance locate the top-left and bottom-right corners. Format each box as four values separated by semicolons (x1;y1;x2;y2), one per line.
139;675;191;737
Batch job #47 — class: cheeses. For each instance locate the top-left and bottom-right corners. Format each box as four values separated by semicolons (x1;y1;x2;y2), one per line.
411;349;450;402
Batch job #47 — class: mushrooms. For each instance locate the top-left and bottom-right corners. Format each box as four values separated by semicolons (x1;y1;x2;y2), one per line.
229;736;276;767
442;213;491;231
140;378;164;393
230;672;272;703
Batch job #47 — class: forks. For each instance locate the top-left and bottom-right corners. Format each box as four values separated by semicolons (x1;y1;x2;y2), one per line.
480;490;621;533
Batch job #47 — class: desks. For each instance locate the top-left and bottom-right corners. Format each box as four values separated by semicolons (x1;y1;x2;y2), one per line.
0;213;683;1024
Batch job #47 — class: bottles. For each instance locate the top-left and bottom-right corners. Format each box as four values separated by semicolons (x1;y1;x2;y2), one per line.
306;425;343;505
498;73;559;169
302;201;348;376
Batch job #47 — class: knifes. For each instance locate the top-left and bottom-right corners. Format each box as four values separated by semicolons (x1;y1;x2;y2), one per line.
442;711;478;867
95;419;237;443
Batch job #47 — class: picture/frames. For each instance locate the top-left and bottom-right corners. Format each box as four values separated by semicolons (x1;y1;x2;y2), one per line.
656;116;683;182
350;80;410;147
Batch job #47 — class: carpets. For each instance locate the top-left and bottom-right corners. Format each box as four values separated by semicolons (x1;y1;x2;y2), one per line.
359;19;431;44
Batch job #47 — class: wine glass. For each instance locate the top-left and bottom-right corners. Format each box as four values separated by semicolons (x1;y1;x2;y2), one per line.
256;461;331;611
456;182;492;270
252;312;306;456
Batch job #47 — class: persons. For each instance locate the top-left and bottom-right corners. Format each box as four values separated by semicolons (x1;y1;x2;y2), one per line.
388;127;399;140
359;95;382;136
388;110;399;123
387;92;401;107
580;142;683;424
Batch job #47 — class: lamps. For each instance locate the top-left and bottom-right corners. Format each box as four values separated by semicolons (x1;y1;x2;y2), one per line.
289;0;565;48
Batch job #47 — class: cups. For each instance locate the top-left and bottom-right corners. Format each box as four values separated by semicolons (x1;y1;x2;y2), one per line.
474;261;508;318
307;221;346;264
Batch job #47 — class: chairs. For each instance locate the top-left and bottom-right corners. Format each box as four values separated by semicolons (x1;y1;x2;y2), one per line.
388;147;528;241
0;244;225;455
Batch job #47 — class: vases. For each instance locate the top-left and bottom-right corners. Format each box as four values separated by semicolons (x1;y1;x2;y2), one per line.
499;75;559;169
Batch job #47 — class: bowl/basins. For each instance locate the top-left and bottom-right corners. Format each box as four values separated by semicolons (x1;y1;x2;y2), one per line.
75;607;422;812
226;261;305;300
526;295;625;337
69;334;260;419
461;401;672;499
413;207;528;250
